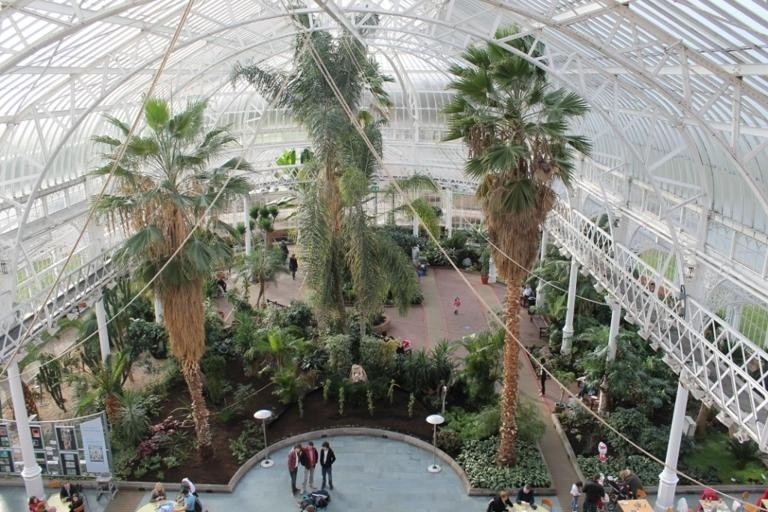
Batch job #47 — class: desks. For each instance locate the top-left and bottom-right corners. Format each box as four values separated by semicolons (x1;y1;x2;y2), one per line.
502;498;729;512
42;493;71;512
139;501;187;512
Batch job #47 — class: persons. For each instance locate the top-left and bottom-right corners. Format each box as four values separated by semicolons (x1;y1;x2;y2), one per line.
67;493;84;512
280;240;287;264
173;485;196;511
521;283;533;309
319;441;336;489
453;296;461;314
415;261;426;283
493;491;514;512
597;472;606;511
300;441;318;493
28;495;57;511
582;473;605;512
289;254;298;278
217;272;228;292
381;331;388;341
60;481;77;499
299;489;330;512
537;357;546;396
569;481;584;512
174;476;198;502
619;468;643;499
516;482;534;504
288;444;302;495
148;481;166;502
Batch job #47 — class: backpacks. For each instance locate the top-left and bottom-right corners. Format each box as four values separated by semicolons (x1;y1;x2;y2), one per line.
194;498;202;512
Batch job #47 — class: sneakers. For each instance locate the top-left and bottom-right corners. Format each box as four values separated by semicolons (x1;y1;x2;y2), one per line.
293;483;333;496
